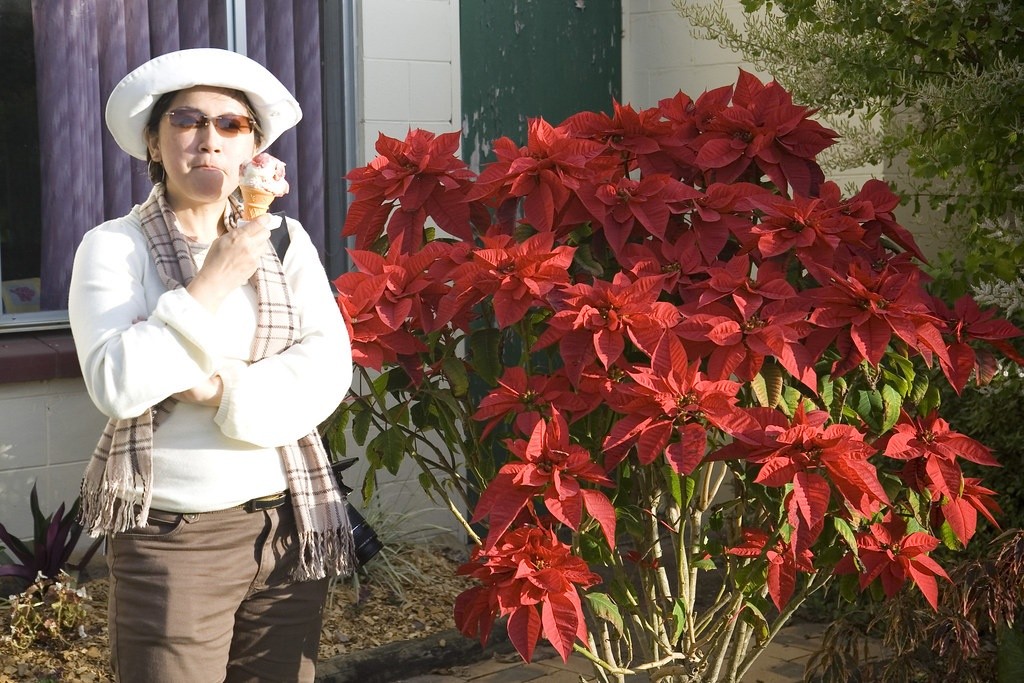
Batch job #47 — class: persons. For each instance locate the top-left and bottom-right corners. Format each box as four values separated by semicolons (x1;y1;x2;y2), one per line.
68;48;354;683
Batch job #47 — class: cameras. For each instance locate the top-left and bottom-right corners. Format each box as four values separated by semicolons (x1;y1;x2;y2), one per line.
331;457;384;571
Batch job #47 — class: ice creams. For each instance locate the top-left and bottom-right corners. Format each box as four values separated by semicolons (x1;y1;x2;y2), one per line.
238;153;290;221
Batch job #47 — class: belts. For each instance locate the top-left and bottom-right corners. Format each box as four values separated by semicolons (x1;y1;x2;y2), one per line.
203;493;289;513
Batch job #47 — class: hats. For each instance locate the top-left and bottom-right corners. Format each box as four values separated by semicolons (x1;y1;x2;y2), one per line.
105;48;304;161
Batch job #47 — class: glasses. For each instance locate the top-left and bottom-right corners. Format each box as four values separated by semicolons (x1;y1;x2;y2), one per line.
159;106;260;134
